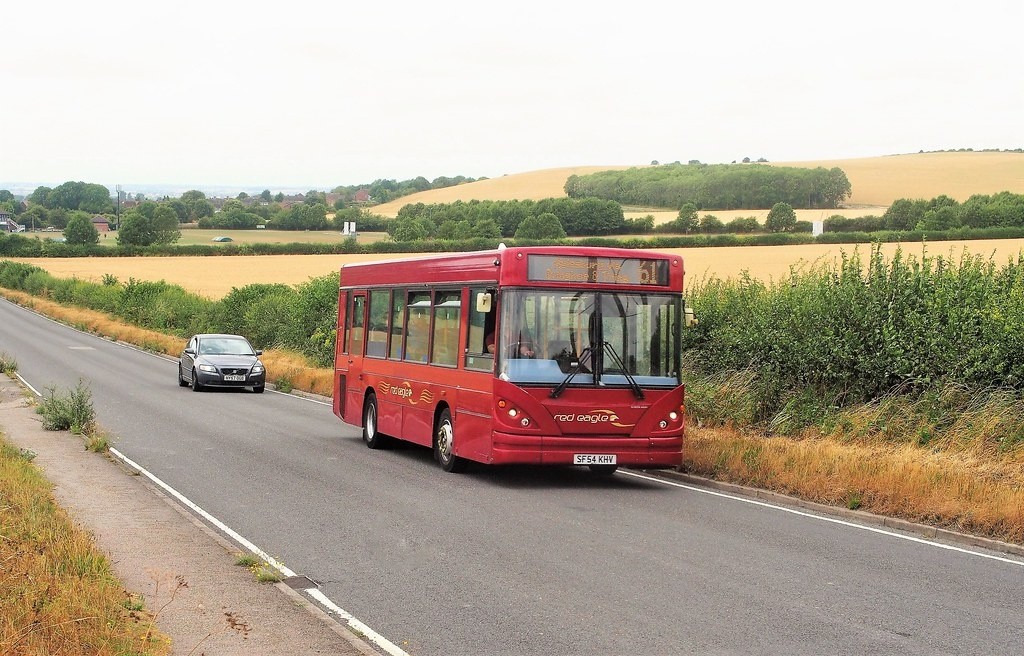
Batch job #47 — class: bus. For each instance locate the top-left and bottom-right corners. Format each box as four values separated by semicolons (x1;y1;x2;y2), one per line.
333;243;699;475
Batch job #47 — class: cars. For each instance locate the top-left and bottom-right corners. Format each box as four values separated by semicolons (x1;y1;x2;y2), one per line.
178;334;267;392
212;237;232;242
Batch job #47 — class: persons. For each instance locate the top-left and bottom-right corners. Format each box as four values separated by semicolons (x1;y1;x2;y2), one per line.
485;311;535;358
